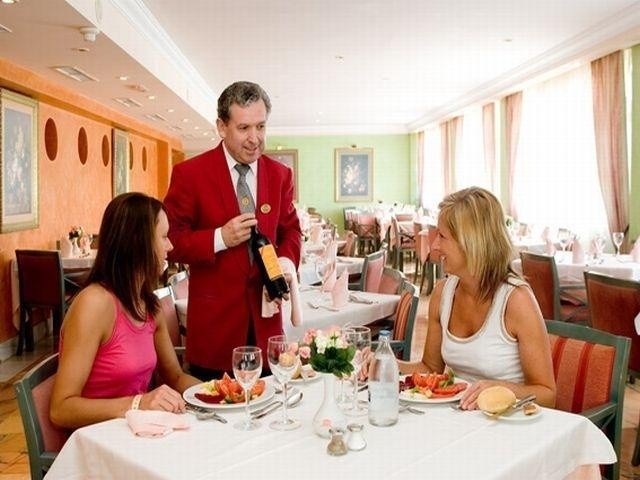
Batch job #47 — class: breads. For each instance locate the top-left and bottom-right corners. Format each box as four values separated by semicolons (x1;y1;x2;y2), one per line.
477;386;516;415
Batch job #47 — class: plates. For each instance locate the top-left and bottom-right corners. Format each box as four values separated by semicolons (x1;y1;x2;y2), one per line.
399;373;472;405
182;379;276;411
483;400;544;421
288;362;320;383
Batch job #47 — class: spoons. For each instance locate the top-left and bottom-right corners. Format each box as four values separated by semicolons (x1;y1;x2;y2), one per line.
185;405;216;421
398;404;410;414
251;390;304;422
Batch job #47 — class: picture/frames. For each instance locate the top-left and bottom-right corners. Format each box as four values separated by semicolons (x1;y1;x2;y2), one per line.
262;148;298;205
111;126;130;200
0;86;38;235
334;147;374;203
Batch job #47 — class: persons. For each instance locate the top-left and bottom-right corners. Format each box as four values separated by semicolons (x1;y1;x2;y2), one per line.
357;182;558;411
162;81;302;382
47;193;203;431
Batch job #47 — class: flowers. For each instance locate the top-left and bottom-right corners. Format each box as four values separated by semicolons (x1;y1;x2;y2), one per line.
65;226;81;243
297;324;358;380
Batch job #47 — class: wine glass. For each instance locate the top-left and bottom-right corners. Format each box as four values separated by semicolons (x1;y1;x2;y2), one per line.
611;231;625;262
342;325;369;418
83;233;93;253
592;234;607;261
557;226;572;262
267;333;301;431
314;259;337;302
231;345;264;432
319;230;332;257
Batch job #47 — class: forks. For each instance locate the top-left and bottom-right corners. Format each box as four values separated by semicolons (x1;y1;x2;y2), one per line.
307;300;339;312
249;387;299;416
398;399;425;415
187;403;229;425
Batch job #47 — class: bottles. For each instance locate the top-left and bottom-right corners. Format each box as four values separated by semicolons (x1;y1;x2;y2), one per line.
326;429;348;457
367;329;400;428
347;424;367;452
250;225;291;303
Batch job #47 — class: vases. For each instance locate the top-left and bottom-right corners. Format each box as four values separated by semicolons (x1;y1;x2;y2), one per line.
313;373;348;438
70;239;81;257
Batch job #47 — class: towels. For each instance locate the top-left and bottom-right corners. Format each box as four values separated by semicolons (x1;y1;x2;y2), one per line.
300;209;327;241
570;235;586;264
126;408;191;437
546;237;557;256
261;255;301;328
332;267;350;308
323;243;337;266
415;206;424;222
322;262;337;293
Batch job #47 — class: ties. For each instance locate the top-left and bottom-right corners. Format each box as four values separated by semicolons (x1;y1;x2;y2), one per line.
234;163;256;260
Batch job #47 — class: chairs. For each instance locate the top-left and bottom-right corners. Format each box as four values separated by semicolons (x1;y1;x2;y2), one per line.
582;270;639;383
146;287;185;383
12;347;70;480
521;251;592;326
368;282;419;361
16;247;70;353
296;207;385;291
544;315;631;478
167;269;189;346
341;205;444;297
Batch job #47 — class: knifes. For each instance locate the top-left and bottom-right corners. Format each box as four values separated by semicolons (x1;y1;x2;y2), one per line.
488;393;538;421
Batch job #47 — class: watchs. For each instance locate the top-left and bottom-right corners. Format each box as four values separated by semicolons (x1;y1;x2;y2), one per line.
130;391;144;411
